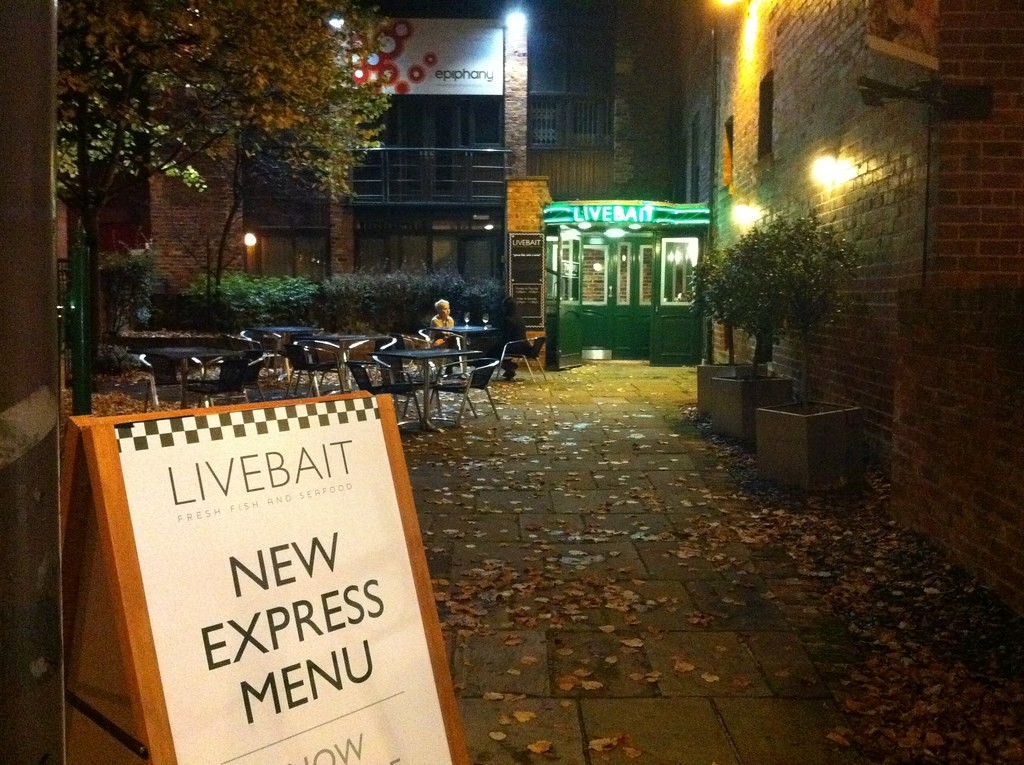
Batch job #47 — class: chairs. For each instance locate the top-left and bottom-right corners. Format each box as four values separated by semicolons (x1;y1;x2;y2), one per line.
124;325;547;413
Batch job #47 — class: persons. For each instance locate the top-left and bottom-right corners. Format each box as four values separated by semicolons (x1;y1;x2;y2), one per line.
470;297;532;389
429;298;454;370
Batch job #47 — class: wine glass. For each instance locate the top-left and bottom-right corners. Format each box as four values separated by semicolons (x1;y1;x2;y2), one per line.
482;312;489;330
463;312;470;329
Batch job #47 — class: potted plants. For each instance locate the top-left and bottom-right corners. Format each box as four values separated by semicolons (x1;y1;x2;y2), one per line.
688;222;881;493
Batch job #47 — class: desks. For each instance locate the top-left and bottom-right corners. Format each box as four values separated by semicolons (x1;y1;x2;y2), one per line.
365;347;480;430
430;324;498;380
250;324;325;389
294;332;391;392
127;347;251;411
